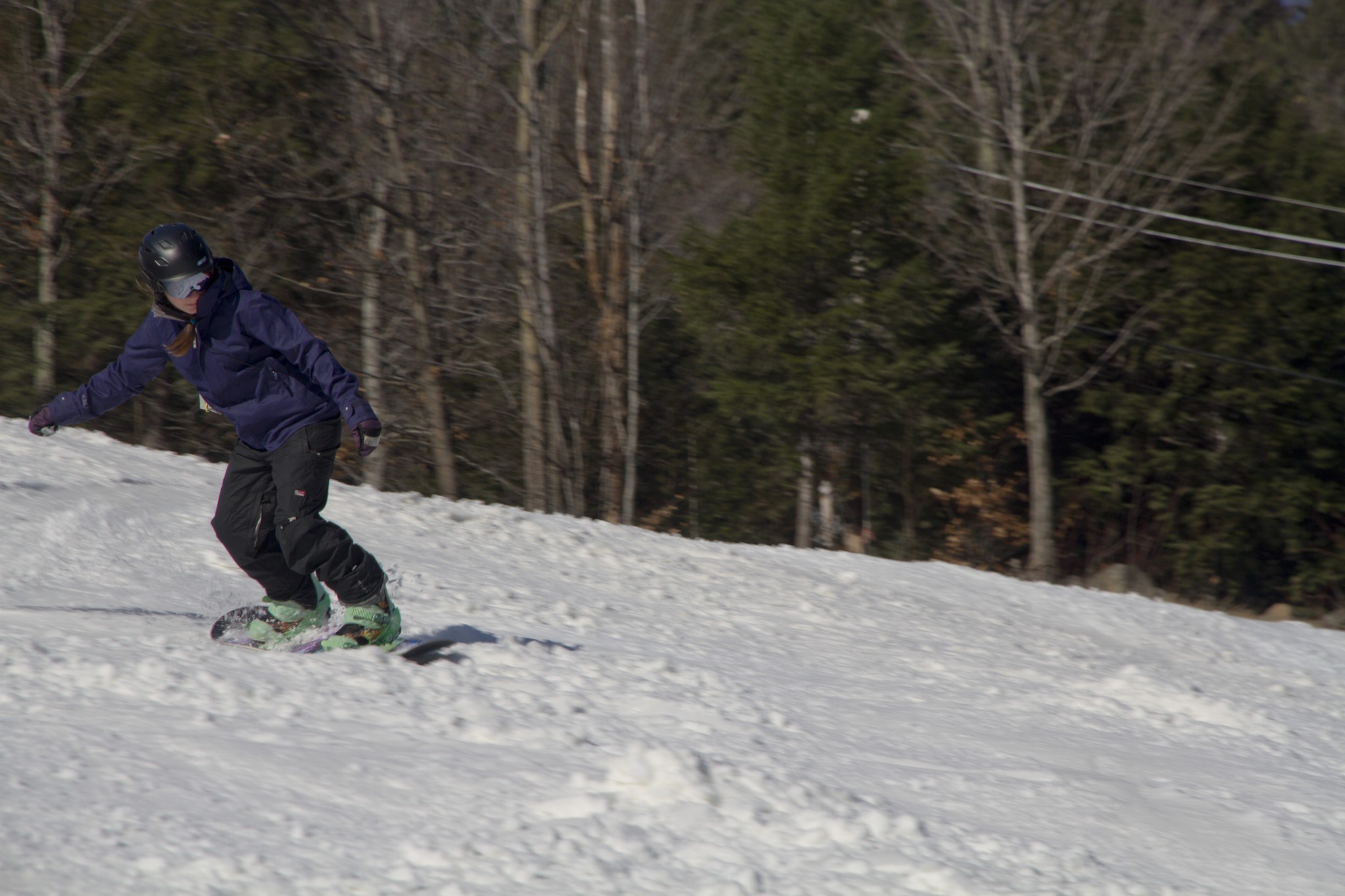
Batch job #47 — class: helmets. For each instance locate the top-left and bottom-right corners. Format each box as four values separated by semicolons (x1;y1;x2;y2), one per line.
138;224;214;293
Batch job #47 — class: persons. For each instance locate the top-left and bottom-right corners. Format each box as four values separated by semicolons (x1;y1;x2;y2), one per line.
26;223;403;650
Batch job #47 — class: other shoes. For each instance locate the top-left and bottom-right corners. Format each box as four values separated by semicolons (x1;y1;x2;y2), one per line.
323;586;402;652
250;588;333;643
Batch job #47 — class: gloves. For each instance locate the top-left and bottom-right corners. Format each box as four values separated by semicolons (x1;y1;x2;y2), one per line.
353;419;383;459
27;408;59;437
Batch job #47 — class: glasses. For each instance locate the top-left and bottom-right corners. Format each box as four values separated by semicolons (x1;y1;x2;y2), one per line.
158;271;213;299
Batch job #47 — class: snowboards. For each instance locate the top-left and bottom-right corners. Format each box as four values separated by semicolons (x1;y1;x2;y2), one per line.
209;603;457;659
